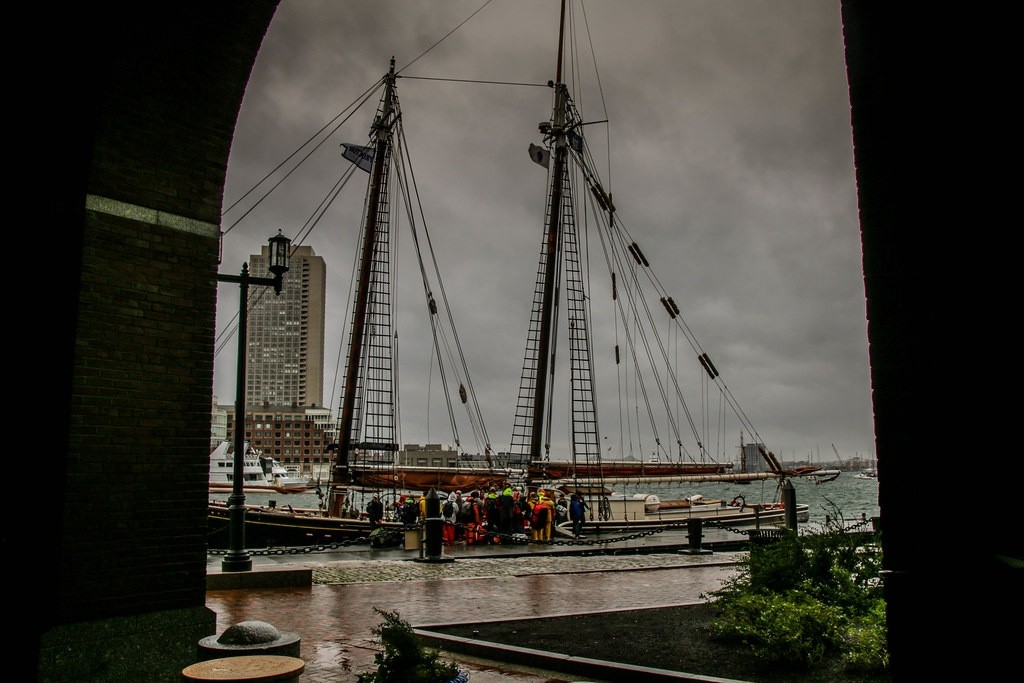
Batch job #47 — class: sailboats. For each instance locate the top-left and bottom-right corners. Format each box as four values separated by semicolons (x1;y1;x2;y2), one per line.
205;0;810;549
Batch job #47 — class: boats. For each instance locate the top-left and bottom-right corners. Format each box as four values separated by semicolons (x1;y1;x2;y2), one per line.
209;440;313;494
852;468;878;480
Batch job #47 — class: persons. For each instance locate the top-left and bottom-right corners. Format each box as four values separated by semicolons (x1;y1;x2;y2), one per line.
366;487;590;549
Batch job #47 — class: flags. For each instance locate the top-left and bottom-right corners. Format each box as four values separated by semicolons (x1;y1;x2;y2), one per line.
566;129;584;155
527;143;551;169
340;142;375;172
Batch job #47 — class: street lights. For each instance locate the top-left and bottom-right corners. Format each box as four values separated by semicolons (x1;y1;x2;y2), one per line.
217;228;291;572
